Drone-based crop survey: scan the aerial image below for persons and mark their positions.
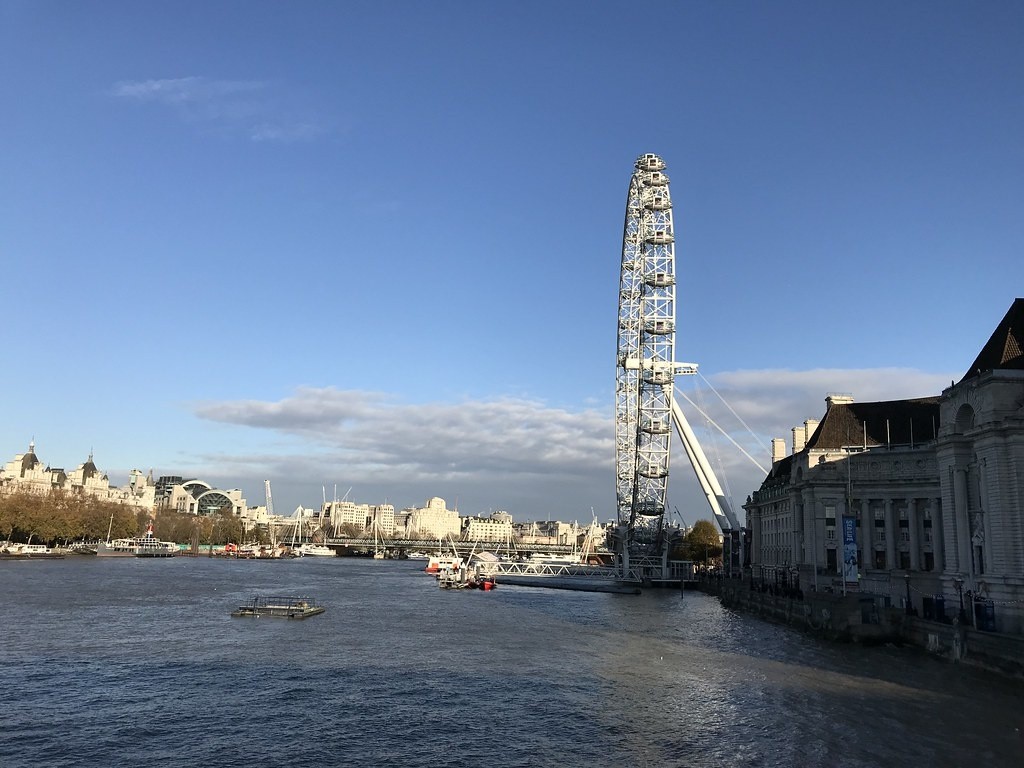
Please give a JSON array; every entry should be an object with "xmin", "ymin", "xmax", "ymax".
[{"xmin": 440, "ymin": 569, "xmax": 446, "ymax": 583}]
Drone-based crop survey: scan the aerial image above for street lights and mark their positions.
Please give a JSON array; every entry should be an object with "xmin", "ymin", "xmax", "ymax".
[
  {"xmin": 953, "ymin": 578, "xmax": 969, "ymax": 626},
  {"xmin": 748, "ymin": 564, "xmax": 794, "ymax": 599},
  {"xmin": 904, "ymin": 575, "xmax": 914, "ymax": 619}
]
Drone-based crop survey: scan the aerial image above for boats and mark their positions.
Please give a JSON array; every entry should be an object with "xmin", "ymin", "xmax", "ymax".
[
  {"xmin": 0, "ymin": 544, "xmax": 52, "ymax": 555},
  {"xmin": 425, "ymin": 554, "xmax": 464, "ymax": 575},
  {"xmin": 230, "ymin": 542, "xmax": 337, "ymax": 559},
  {"xmin": 97, "ymin": 537, "xmax": 181, "ymax": 557}
]
